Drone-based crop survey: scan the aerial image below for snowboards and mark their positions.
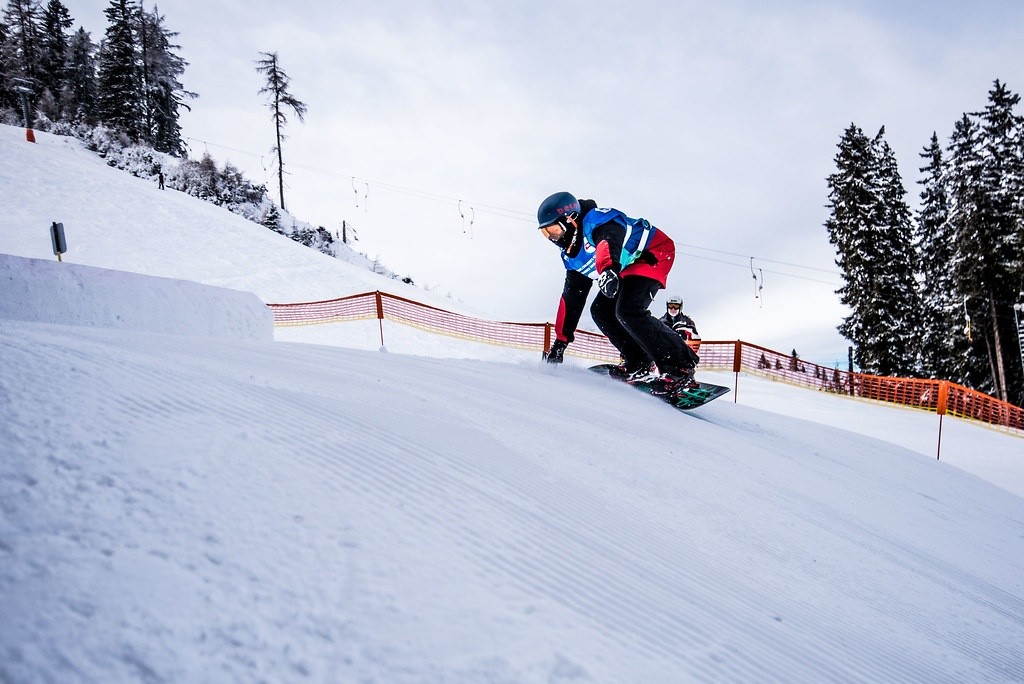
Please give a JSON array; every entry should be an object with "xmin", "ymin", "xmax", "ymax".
[{"xmin": 588, "ymin": 364, "xmax": 730, "ymax": 410}]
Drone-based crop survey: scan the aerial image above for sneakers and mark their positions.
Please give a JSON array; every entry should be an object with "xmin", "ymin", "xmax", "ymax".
[
  {"xmin": 610, "ymin": 362, "xmax": 655, "ymax": 384},
  {"xmin": 650, "ymin": 366, "xmax": 696, "ymax": 396}
]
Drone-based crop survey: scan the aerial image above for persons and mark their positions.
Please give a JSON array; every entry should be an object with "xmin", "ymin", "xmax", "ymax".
[
  {"xmin": 158, "ymin": 173, "xmax": 164, "ymax": 190},
  {"xmin": 536, "ymin": 191, "xmax": 700, "ymax": 398},
  {"xmin": 658, "ymin": 294, "xmax": 703, "ymax": 353}
]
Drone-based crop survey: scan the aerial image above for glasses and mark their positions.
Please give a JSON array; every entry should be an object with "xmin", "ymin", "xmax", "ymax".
[{"xmin": 668, "ymin": 304, "xmax": 680, "ymax": 309}]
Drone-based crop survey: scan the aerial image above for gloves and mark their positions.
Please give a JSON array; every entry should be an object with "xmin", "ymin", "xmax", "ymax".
[
  {"xmin": 597, "ymin": 269, "xmax": 619, "ymax": 298},
  {"xmin": 546, "ymin": 339, "xmax": 567, "ymax": 363}
]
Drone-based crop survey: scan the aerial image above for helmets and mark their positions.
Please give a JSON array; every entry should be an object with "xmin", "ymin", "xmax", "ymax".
[
  {"xmin": 665, "ymin": 296, "xmax": 683, "ymax": 315},
  {"xmin": 536, "ymin": 191, "xmax": 581, "ymax": 248}
]
